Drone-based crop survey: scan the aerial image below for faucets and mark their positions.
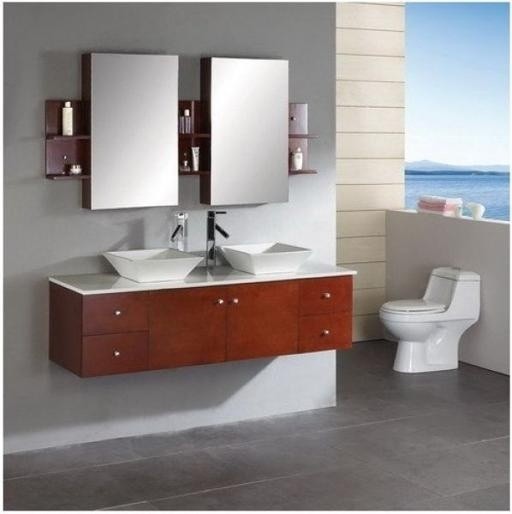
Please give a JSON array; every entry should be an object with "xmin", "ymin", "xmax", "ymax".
[
  {"xmin": 204, "ymin": 208, "xmax": 230, "ymax": 269},
  {"xmin": 171, "ymin": 210, "xmax": 190, "ymax": 251}
]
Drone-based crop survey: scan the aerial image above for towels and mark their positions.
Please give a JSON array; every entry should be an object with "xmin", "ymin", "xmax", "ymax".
[{"xmin": 415, "ymin": 194, "xmax": 463, "ymax": 219}]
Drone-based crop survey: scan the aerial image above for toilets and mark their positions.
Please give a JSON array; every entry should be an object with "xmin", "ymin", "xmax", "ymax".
[{"xmin": 377, "ymin": 268, "xmax": 481, "ymax": 374}]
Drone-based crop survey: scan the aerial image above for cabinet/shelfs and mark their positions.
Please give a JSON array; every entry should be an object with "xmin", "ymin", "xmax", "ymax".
[
  {"xmin": 47, "ymin": 276, "xmax": 354, "ymax": 378},
  {"xmin": 179, "ymin": 99, "xmax": 207, "ymax": 176},
  {"xmin": 289, "ymin": 102, "xmax": 318, "ymax": 175},
  {"xmin": 45, "ymin": 98, "xmax": 91, "ymax": 181}
]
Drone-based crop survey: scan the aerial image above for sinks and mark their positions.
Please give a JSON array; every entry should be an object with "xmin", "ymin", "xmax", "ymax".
[
  {"xmin": 102, "ymin": 246, "xmax": 204, "ymax": 285},
  {"xmin": 215, "ymin": 240, "xmax": 314, "ymax": 274}
]
